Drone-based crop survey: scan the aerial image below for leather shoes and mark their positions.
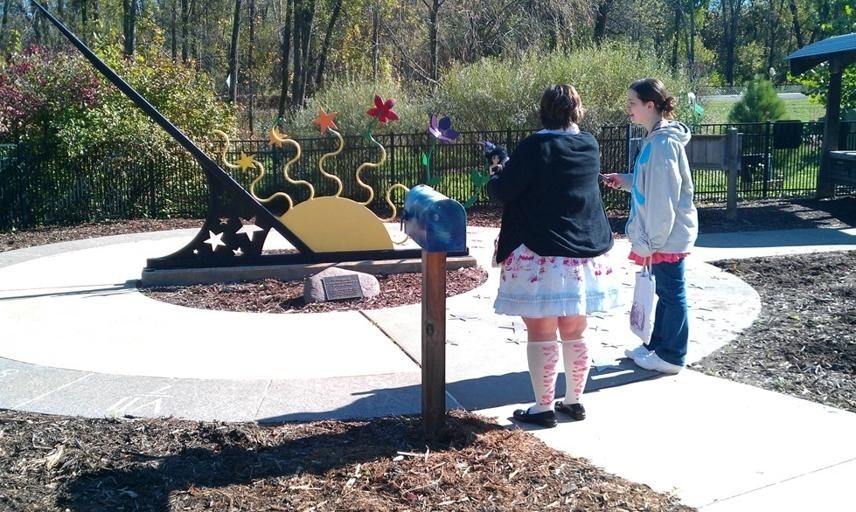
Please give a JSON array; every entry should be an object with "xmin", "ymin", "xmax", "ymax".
[
  {"xmin": 554, "ymin": 400, "xmax": 586, "ymax": 421},
  {"xmin": 513, "ymin": 408, "xmax": 558, "ymax": 428}
]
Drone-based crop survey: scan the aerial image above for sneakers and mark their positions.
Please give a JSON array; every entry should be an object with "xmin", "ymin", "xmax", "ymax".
[{"xmin": 624, "ymin": 345, "xmax": 684, "ymax": 375}]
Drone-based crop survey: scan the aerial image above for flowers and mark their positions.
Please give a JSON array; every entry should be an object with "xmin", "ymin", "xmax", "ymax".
[
  {"xmin": 418, "ymin": 112, "xmax": 462, "ymax": 188},
  {"xmin": 355, "ymin": 93, "xmax": 400, "ymax": 206}
]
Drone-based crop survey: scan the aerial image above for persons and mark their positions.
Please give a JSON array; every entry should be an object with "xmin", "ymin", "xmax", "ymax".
[
  {"xmin": 486, "ymin": 149, "xmax": 504, "ymax": 176},
  {"xmin": 485, "ymin": 82, "xmax": 617, "ymax": 427},
  {"xmin": 603, "ymin": 75, "xmax": 701, "ymax": 374}
]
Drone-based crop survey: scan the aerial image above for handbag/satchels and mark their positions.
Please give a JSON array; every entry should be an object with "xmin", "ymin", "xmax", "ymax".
[{"xmin": 629, "ymin": 255, "xmax": 657, "ymax": 346}]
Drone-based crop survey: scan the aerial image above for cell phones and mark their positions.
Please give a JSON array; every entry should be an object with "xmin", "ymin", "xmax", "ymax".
[{"xmin": 599, "ymin": 173, "xmax": 611, "ymax": 182}]
